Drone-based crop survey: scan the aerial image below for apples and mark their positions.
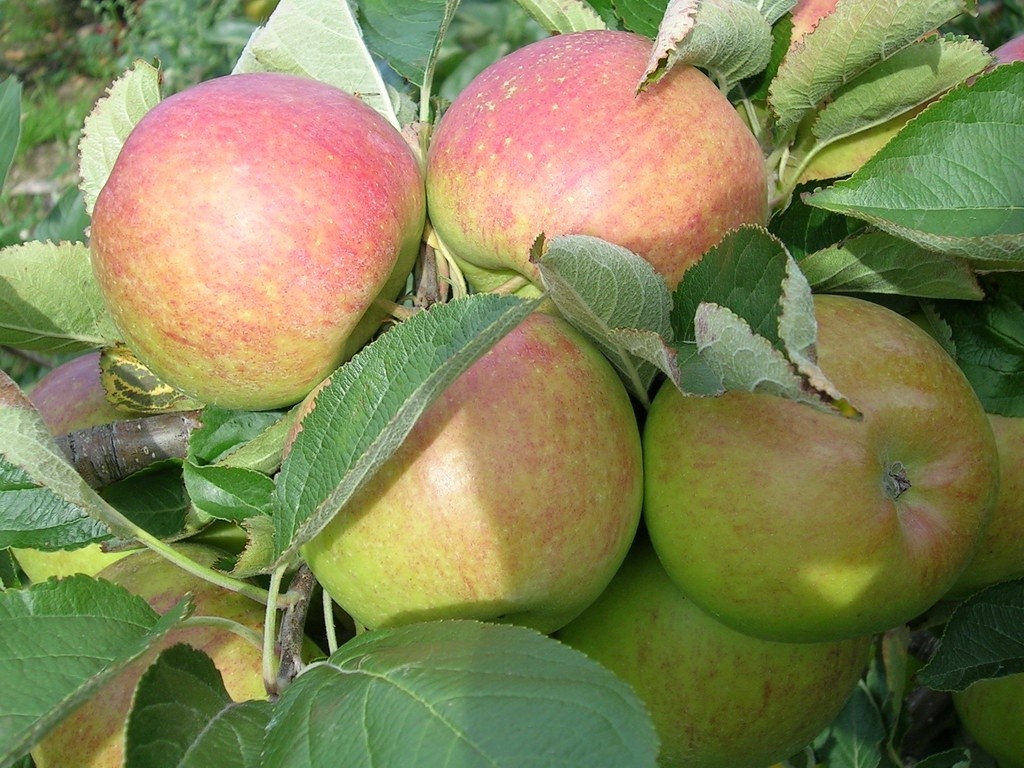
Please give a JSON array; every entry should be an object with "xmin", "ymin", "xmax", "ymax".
[{"xmin": 1, "ymin": 0, "xmax": 1024, "ymax": 768}]
